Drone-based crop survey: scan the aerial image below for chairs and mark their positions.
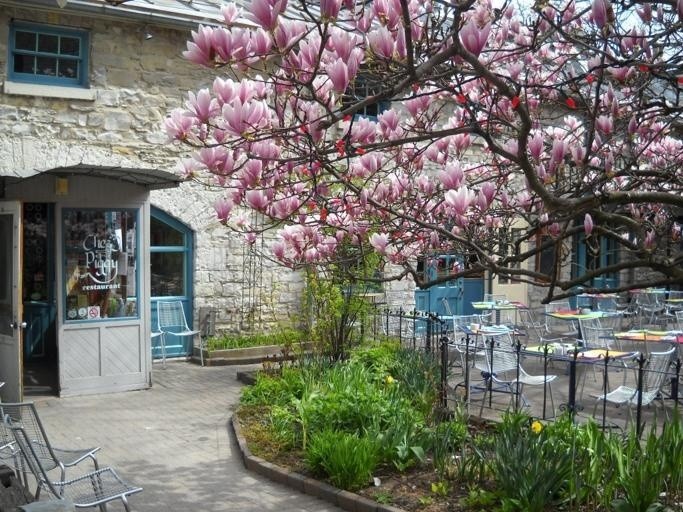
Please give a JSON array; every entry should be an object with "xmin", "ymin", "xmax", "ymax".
[
  {"xmin": 545, "ymin": 288, "xmax": 683, "ymax": 432},
  {"xmin": 439, "ymin": 294, "xmax": 582, "ymax": 418},
  {"xmin": 151, "ymin": 331, "xmax": 166, "ymax": 367},
  {"xmin": 157, "ymin": 299, "xmax": 204, "ymax": 368},
  {"xmin": 0, "ymin": 400, "xmax": 144, "ymax": 512}
]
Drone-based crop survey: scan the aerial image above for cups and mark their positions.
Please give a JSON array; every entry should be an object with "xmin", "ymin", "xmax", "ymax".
[{"xmin": 470, "ymin": 323, "xmax": 480, "ymax": 331}]
